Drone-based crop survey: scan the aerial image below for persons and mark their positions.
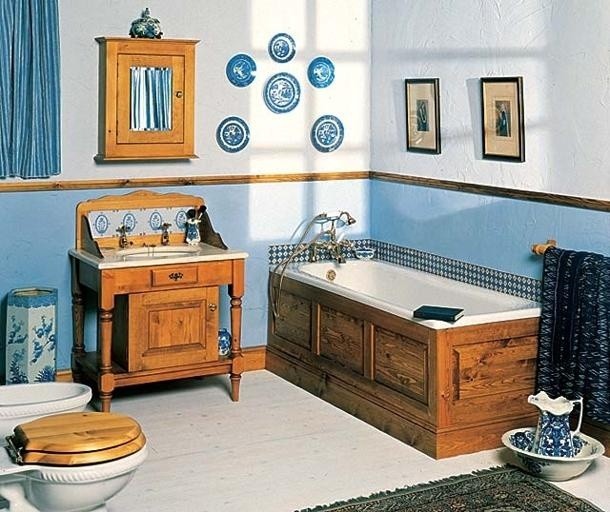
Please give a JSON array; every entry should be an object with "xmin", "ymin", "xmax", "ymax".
[
  {"xmin": 499, "ymin": 103, "xmax": 509, "ymax": 136},
  {"xmin": 419, "ymin": 102, "xmax": 427, "ymax": 131}
]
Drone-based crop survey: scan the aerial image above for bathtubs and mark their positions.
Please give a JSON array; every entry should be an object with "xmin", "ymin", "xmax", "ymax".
[{"xmin": 297, "ymin": 253, "xmax": 542, "ymax": 331}]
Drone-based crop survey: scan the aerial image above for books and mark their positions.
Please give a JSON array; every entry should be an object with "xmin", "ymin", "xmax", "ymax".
[{"xmin": 413, "ymin": 305, "xmax": 465, "ymax": 322}]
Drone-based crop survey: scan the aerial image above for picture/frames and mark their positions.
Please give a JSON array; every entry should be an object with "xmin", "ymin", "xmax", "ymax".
[
  {"xmin": 479, "ymin": 76, "xmax": 527, "ymax": 165},
  {"xmin": 403, "ymin": 77, "xmax": 442, "ymax": 155}
]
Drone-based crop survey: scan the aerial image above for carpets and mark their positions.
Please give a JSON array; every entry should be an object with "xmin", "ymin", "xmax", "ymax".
[{"xmin": 292, "ymin": 461, "xmax": 604, "ymax": 512}]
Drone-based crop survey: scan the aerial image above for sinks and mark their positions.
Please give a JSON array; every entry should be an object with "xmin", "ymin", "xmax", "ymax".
[
  {"xmin": 120, "ymin": 247, "xmax": 200, "ymax": 260},
  {"xmin": 0, "ymin": 381, "xmax": 93, "ymax": 442}
]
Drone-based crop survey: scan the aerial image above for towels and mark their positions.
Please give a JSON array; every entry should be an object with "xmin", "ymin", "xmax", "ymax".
[{"xmin": 534, "ymin": 245, "xmax": 610, "ymax": 434}]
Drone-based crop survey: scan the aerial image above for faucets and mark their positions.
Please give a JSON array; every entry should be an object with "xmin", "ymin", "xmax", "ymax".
[
  {"xmin": 116, "ymin": 225, "xmax": 127, "ymax": 248},
  {"xmin": 327, "ymin": 240, "xmax": 347, "ymax": 264},
  {"xmin": 158, "ymin": 222, "xmax": 172, "ymax": 244}
]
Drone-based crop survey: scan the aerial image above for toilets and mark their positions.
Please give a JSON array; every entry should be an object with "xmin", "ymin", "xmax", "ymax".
[{"xmin": 0, "ymin": 411, "xmax": 148, "ymax": 510}]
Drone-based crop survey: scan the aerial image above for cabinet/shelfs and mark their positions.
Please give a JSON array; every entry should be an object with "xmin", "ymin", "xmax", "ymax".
[
  {"xmin": 93, "ymin": 35, "xmax": 201, "ymax": 163},
  {"xmin": 70, "ymin": 257, "xmax": 246, "ymax": 413}
]
